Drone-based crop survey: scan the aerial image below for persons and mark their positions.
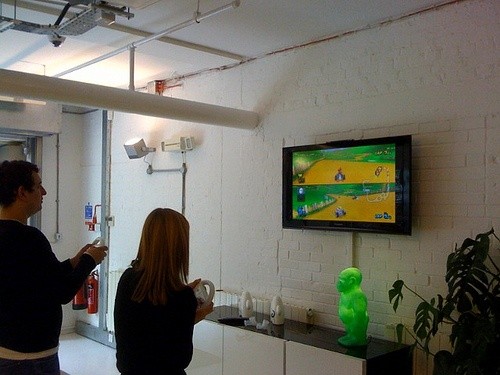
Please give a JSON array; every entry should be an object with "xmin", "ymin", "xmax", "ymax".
[
  {"xmin": 0, "ymin": 160, "xmax": 107, "ymax": 374},
  {"xmin": 335, "ymin": 267, "xmax": 370, "ymax": 346},
  {"xmin": 115, "ymin": 207, "xmax": 214, "ymax": 375}
]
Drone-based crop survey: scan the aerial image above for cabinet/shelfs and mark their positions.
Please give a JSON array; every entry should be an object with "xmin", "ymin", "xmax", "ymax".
[
  {"xmin": 223, "ymin": 323, "xmax": 287, "ymax": 375},
  {"xmin": 184, "ymin": 320, "xmax": 223, "ymax": 375},
  {"xmin": 285, "ymin": 340, "xmax": 364, "ymax": 375}
]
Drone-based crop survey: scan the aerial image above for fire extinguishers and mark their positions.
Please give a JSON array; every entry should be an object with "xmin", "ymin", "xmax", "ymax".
[{"xmin": 86, "ymin": 271, "xmax": 98, "ymax": 313}]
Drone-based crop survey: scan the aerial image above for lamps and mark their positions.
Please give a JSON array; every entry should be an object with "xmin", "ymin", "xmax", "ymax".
[{"xmin": 123, "ymin": 136, "xmax": 157, "ymax": 159}]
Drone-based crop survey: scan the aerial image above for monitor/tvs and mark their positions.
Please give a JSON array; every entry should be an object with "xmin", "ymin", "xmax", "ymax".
[{"xmin": 282, "ymin": 135, "xmax": 412, "ymax": 236}]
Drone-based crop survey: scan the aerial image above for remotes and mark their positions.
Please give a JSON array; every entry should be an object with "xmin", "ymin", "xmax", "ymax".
[{"xmin": 218, "ymin": 319, "xmax": 252, "ymax": 327}]
